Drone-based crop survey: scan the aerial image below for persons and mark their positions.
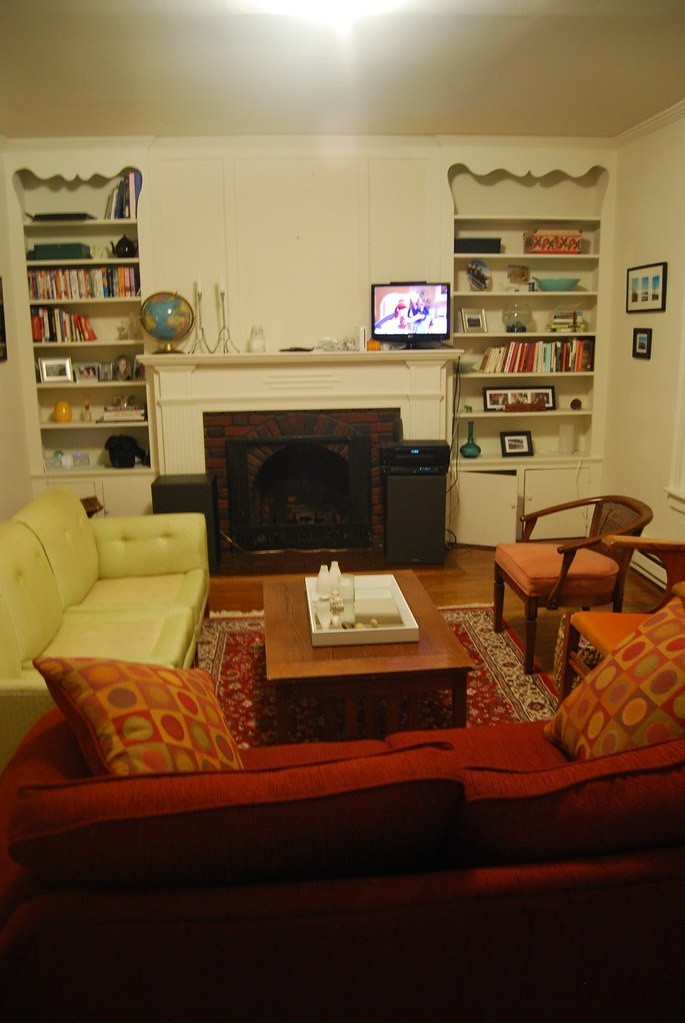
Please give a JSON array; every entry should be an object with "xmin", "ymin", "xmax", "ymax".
[{"xmin": 115, "ymin": 356, "xmax": 132, "ymax": 381}]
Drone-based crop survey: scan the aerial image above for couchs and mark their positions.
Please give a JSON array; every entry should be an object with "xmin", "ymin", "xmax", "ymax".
[
  {"xmin": 0, "ymin": 705, "xmax": 685, "ymax": 1023},
  {"xmin": 0, "ymin": 483, "xmax": 210, "ymax": 774}
]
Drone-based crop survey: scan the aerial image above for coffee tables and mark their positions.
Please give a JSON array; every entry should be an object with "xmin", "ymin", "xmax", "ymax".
[{"xmin": 261, "ymin": 569, "xmax": 473, "ymax": 744}]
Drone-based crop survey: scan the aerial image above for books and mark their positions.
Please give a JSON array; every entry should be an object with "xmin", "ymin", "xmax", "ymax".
[
  {"xmin": 485, "ymin": 341, "xmax": 583, "ymax": 373},
  {"xmin": 27, "ymin": 266, "xmax": 135, "ymax": 299},
  {"xmin": 31, "ymin": 306, "xmax": 97, "ymax": 343},
  {"xmin": 104, "ymin": 174, "xmax": 129, "ymax": 220},
  {"xmin": 545, "ymin": 311, "xmax": 585, "ymax": 332}
]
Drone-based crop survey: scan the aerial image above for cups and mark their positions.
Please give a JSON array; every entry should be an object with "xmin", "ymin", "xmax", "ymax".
[{"xmin": 338, "ymin": 574, "xmax": 355, "ymax": 601}]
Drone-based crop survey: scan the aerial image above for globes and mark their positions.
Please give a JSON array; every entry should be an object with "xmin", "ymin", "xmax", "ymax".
[{"xmin": 136, "ymin": 290, "xmax": 194, "ymax": 354}]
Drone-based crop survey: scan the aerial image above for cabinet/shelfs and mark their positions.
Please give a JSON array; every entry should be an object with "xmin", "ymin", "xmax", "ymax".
[
  {"xmin": 452, "ymin": 212, "xmax": 602, "ymax": 467},
  {"xmin": 23, "ymin": 218, "xmax": 158, "ymax": 477},
  {"xmin": 32, "ymin": 475, "xmax": 155, "ymax": 518},
  {"xmin": 450, "ymin": 466, "xmax": 592, "ymax": 547}
]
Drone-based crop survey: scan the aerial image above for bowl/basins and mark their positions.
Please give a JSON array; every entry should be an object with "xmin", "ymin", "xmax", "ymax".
[{"xmin": 536, "ymin": 278, "xmax": 581, "ymax": 291}]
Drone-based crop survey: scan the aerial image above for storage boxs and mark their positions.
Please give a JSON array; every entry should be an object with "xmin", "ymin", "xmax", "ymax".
[
  {"xmin": 522, "ymin": 228, "xmax": 584, "ymax": 254},
  {"xmin": 34, "ymin": 243, "xmax": 91, "ymax": 260}
]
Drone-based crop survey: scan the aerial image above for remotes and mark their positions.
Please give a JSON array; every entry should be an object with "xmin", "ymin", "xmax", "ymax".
[{"xmin": 280, "ymin": 346, "xmax": 314, "ymax": 352}]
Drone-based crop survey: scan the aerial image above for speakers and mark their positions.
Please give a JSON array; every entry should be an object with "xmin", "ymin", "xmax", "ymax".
[
  {"xmin": 387, "ymin": 476, "xmax": 447, "ymax": 566},
  {"xmin": 151, "ymin": 474, "xmax": 225, "ymax": 575}
]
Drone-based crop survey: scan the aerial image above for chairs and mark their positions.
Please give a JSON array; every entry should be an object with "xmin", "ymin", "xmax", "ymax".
[
  {"xmin": 556, "ymin": 534, "xmax": 685, "ymax": 708},
  {"xmin": 492, "ymin": 493, "xmax": 653, "ymax": 675}
]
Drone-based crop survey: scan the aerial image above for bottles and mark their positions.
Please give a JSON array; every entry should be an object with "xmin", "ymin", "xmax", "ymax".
[
  {"xmin": 330, "ymin": 561, "xmax": 342, "ymax": 595},
  {"xmin": 250, "ymin": 326, "xmax": 265, "ymax": 352},
  {"xmin": 502, "ymin": 302, "xmax": 533, "ymax": 332},
  {"xmin": 460, "ymin": 421, "xmax": 481, "ymax": 458},
  {"xmin": 316, "ymin": 565, "xmax": 331, "ymax": 601},
  {"xmin": 120, "ymin": 312, "xmax": 137, "ymax": 340},
  {"xmin": 330, "ymin": 590, "xmax": 343, "ymax": 609},
  {"xmin": 53, "ymin": 450, "xmax": 81, "ymax": 471},
  {"xmin": 54, "ymin": 401, "xmax": 72, "ymax": 423}
]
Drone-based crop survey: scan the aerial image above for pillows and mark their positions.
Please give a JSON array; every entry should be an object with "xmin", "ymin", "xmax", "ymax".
[
  {"xmin": 540, "ymin": 595, "xmax": 685, "ymax": 763},
  {"xmin": 32, "ymin": 655, "xmax": 243, "ymax": 776}
]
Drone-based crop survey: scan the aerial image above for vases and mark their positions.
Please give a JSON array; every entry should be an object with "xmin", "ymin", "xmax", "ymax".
[{"xmin": 460, "ymin": 421, "xmax": 481, "ymax": 458}]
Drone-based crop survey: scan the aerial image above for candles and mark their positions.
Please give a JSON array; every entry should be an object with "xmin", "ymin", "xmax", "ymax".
[
  {"xmin": 218, "ymin": 278, "xmax": 226, "ymax": 294},
  {"xmin": 195, "ymin": 279, "xmax": 202, "ymax": 294}
]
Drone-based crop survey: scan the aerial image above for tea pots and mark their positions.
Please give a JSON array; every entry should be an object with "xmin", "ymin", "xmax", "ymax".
[{"xmin": 110, "ymin": 234, "xmax": 139, "ymax": 258}]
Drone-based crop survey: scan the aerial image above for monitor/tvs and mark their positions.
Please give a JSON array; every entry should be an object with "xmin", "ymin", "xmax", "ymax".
[{"xmin": 372, "ymin": 282, "xmax": 451, "ymax": 350}]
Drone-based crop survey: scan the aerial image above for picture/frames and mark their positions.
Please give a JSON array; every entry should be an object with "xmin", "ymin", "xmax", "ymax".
[
  {"xmin": 499, "ymin": 429, "xmax": 535, "ymax": 458},
  {"xmin": 632, "ymin": 327, "xmax": 652, "ymax": 359},
  {"xmin": 482, "ymin": 386, "xmax": 558, "ymax": 412},
  {"xmin": 626, "ymin": 261, "xmax": 668, "ymax": 314},
  {"xmin": 460, "ymin": 307, "xmax": 487, "ymax": 333},
  {"xmin": 99, "ymin": 361, "xmax": 113, "ymax": 381},
  {"xmin": 73, "ymin": 361, "xmax": 99, "ymax": 383},
  {"xmin": 37, "ymin": 355, "xmax": 75, "ymax": 384}
]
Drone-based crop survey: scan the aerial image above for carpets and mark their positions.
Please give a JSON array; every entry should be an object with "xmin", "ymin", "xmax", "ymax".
[{"xmin": 194, "ymin": 601, "xmax": 563, "ymax": 749}]
